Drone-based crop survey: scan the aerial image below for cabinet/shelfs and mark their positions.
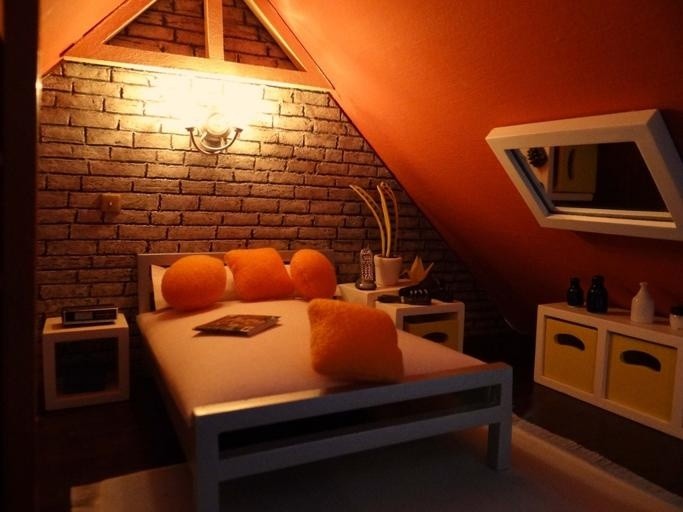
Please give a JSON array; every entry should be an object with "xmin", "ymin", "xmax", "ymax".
[
  {"xmin": 512, "ymin": 146, "xmax": 600, "ymax": 203},
  {"xmin": 533, "ymin": 302, "xmax": 683, "ymax": 438}
]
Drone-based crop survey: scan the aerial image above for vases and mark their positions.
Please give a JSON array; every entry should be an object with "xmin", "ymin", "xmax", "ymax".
[
  {"xmin": 567, "ymin": 277, "xmax": 584, "ymax": 306},
  {"xmin": 586, "ymin": 275, "xmax": 608, "ymax": 314}
]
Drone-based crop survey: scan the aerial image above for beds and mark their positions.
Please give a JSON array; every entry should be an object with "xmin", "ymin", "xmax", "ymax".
[{"xmin": 136, "ymin": 251, "xmax": 513, "ymax": 512}]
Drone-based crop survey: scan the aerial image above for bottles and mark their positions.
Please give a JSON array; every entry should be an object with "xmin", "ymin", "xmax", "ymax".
[{"xmin": 566, "ymin": 274, "xmax": 609, "ymax": 315}]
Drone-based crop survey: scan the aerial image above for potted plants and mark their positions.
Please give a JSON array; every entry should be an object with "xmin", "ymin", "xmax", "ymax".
[{"xmin": 348, "ymin": 182, "xmax": 403, "ymax": 286}]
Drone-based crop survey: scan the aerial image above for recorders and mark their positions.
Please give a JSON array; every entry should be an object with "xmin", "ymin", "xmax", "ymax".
[{"xmin": 61, "ymin": 302, "xmax": 118, "ymax": 326}]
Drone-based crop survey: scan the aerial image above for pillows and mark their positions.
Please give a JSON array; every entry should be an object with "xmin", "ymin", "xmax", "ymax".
[
  {"xmin": 308, "ymin": 297, "xmax": 404, "ymax": 380},
  {"xmin": 225, "ymin": 246, "xmax": 294, "ymax": 303},
  {"xmin": 150, "ymin": 264, "xmax": 343, "ymax": 310},
  {"xmin": 162, "ymin": 256, "xmax": 226, "ymax": 311},
  {"xmin": 290, "ymin": 248, "xmax": 337, "ymax": 301}
]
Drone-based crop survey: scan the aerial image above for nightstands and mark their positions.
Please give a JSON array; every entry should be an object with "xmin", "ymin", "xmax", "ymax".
[
  {"xmin": 338, "ymin": 279, "xmax": 465, "ymax": 354},
  {"xmin": 41, "ymin": 313, "xmax": 129, "ymax": 411}
]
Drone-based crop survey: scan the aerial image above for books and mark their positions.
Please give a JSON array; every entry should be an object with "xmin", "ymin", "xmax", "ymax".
[{"xmin": 191, "ymin": 314, "xmax": 281, "ymax": 338}]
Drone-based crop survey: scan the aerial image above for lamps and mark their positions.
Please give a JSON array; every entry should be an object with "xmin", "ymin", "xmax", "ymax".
[{"xmin": 184, "ymin": 111, "xmax": 244, "ymax": 154}]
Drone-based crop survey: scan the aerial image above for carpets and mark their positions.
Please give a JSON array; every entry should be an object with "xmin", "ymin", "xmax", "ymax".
[{"xmin": 71, "ymin": 407, "xmax": 683, "ymax": 512}]
